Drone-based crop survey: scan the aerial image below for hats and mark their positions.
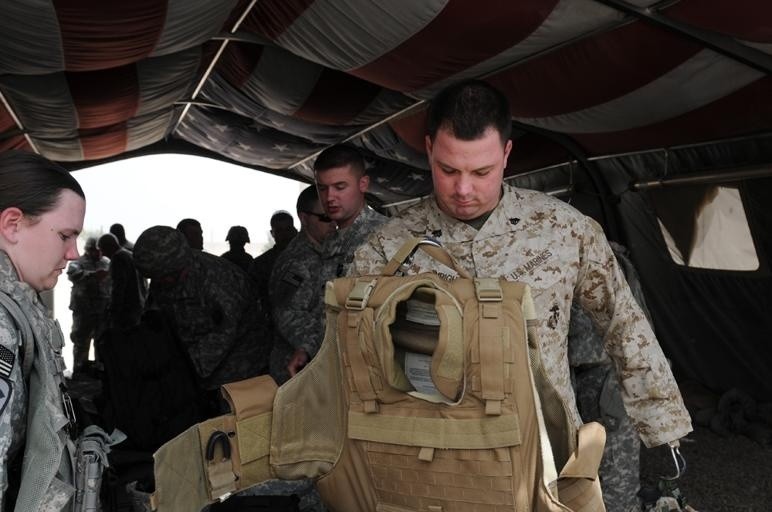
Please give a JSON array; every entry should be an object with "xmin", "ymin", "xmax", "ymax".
[
  {"xmin": 85, "ymin": 238, "xmax": 99, "ymax": 250},
  {"xmin": 132, "ymin": 226, "xmax": 194, "ymax": 276},
  {"xmin": 226, "ymin": 228, "xmax": 250, "ymax": 244}
]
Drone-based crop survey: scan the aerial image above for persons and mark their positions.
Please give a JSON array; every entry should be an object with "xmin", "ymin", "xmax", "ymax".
[
  {"xmin": 343, "ymin": 87, "xmax": 693, "ymax": 512},
  {"xmin": 67, "ymin": 236, "xmax": 112, "ymax": 383},
  {"xmin": 248, "ymin": 210, "xmax": 297, "ymax": 282},
  {"xmin": 218, "ymin": 225, "xmax": 253, "ymax": 270},
  {"xmin": 110, "ymin": 224, "xmax": 134, "ymax": 252},
  {"xmin": 96, "ymin": 234, "xmax": 148, "ymax": 356},
  {"xmin": 0, "ymin": 147, "xmax": 86, "ymax": 512},
  {"xmin": 132, "ymin": 225, "xmax": 264, "ymax": 391},
  {"xmin": 567, "ymin": 237, "xmax": 652, "ymax": 512},
  {"xmin": 278, "ymin": 145, "xmax": 391, "ymax": 377},
  {"xmin": 177, "ymin": 218, "xmax": 203, "ymax": 251},
  {"xmin": 267, "ymin": 184, "xmax": 337, "ymax": 381}
]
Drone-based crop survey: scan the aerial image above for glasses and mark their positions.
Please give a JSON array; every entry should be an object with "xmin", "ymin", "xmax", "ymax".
[{"xmin": 302, "ymin": 210, "xmax": 334, "ymax": 226}]
